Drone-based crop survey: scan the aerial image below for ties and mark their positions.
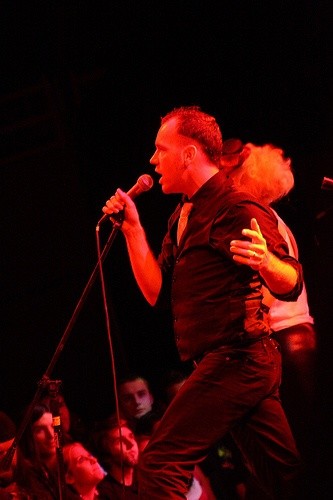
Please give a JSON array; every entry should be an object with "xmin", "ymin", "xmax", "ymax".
[{"xmin": 176, "ymin": 202, "xmax": 192, "ymax": 247}]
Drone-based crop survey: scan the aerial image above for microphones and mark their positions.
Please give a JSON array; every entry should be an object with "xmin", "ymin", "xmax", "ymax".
[{"xmin": 98, "ymin": 174, "xmax": 153, "ymax": 225}]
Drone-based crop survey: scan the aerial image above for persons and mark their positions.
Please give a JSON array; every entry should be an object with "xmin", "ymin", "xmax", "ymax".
[
  {"xmin": 0, "ymin": 373, "xmax": 248, "ymax": 500},
  {"xmin": 101, "ymin": 106, "xmax": 304, "ymax": 500},
  {"xmin": 221, "ymin": 142, "xmax": 320, "ymax": 439}
]
